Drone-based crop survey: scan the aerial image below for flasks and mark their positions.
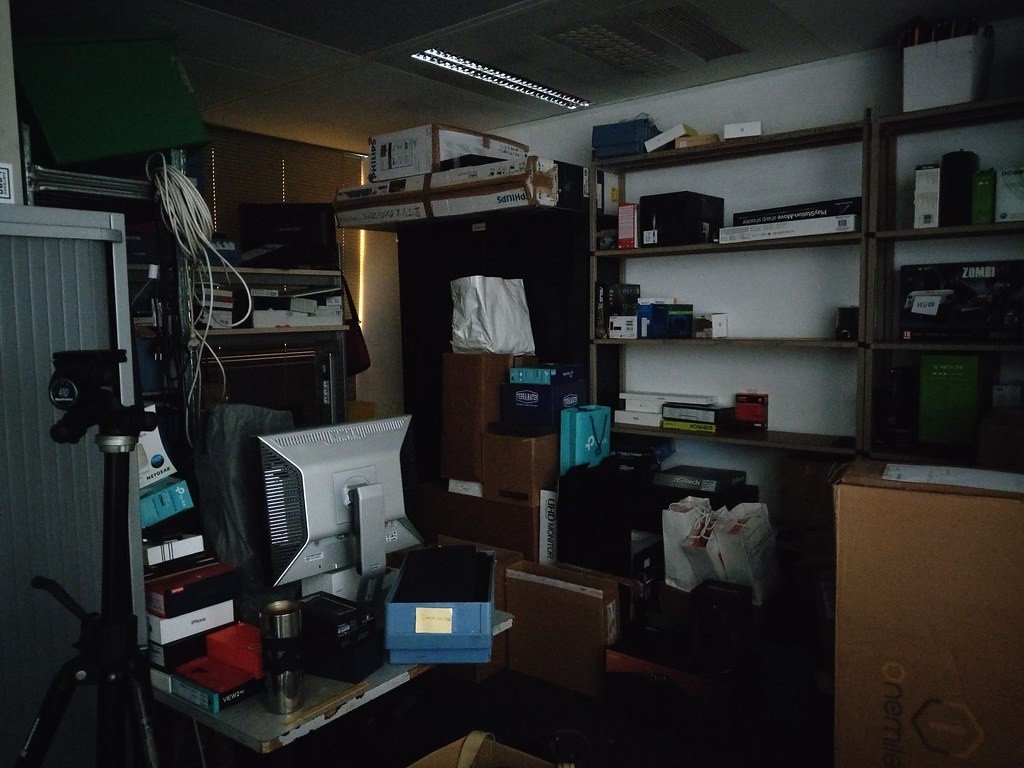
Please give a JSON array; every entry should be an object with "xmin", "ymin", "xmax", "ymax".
[{"xmin": 261, "ymin": 599, "xmax": 303, "ymax": 715}]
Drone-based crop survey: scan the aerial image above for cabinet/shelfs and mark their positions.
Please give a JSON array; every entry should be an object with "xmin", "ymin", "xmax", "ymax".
[
  {"xmin": 586, "ymin": 108, "xmax": 871, "ymax": 457},
  {"xmin": 868, "ymin": 92, "xmax": 1024, "ymax": 467}
]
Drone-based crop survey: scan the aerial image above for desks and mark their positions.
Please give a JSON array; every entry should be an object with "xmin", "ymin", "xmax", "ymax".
[{"xmin": 148, "ymin": 607, "xmax": 517, "ymax": 755}]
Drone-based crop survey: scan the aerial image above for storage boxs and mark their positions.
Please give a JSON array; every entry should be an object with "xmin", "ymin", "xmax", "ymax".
[
  {"xmin": 652, "ymin": 466, "xmax": 747, "ymax": 495},
  {"xmin": 423, "ymin": 488, "xmax": 540, "ymax": 563},
  {"xmin": 384, "ymin": 547, "xmax": 497, "ymax": 665},
  {"xmin": 436, "ymin": 533, "xmax": 523, "ymax": 674},
  {"xmin": 486, "ymin": 360, "xmax": 589, "ymax": 439},
  {"xmin": 971, "ymin": 165, "xmax": 996, "ymax": 226},
  {"xmin": 482, "ymin": 431, "xmax": 560, "ymax": 510},
  {"xmin": 606, "ymin": 623, "xmax": 762, "ymax": 755},
  {"xmin": 536, "ymin": 486, "xmax": 559, "ymax": 564},
  {"xmin": 334, "ymin": 156, "xmax": 619, "ymax": 230},
  {"xmin": 592, "ymin": 111, "xmax": 862, "ymax": 435},
  {"xmin": 830, "ymin": 458, "xmax": 1024, "ymax": 768},
  {"xmin": 684, "ymin": 579, "xmax": 754, "ymax": 652},
  {"xmin": 406, "ymin": 731, "xmax": 555, "ymax": 768},
  {"xmin": 995, "ymin": 166, "xmax": 1024, "ymax": 222},
  {"xmin": 438, "ymin": 352, "xmax": 540, "ymax": 483},
  {"xmin": 136, "ymin": 396, "xmax": 388, "ymax": 713},
  {"xmin": 503, "ymin": 558, "xmax": 621, "ymax": 700},
  {"xmin": 913, "ymin": 163, "xmax": 941, "ymax": 227},
  {"xmin": 899, "ymin": 260, "xmax": 1024, "ymax": 344},
  {"xmin": 367, "ymin": 122, "xmax": 530, "ymax": 184}
]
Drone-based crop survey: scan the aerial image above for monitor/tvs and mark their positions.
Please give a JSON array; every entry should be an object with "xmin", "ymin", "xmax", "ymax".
[{"xmin": 248, "ymin": 407, "xmax": 443, "ymax": 628}]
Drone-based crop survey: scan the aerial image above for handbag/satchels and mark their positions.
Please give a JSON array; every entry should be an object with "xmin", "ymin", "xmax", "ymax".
[
  {"xmin": 662, "ymin": 495, "xmax": 777, "ymax": 605},
  {"xmin": 560, "ymin": 404, "xmax": 611, "ymax": 477},
  {"xmin": 500, "ymin": 383, "xmax": 560, "ymax": 438},
  {"xmin": 450, "ymin": 275, "xmax": 535, "ymax": 357}
]
